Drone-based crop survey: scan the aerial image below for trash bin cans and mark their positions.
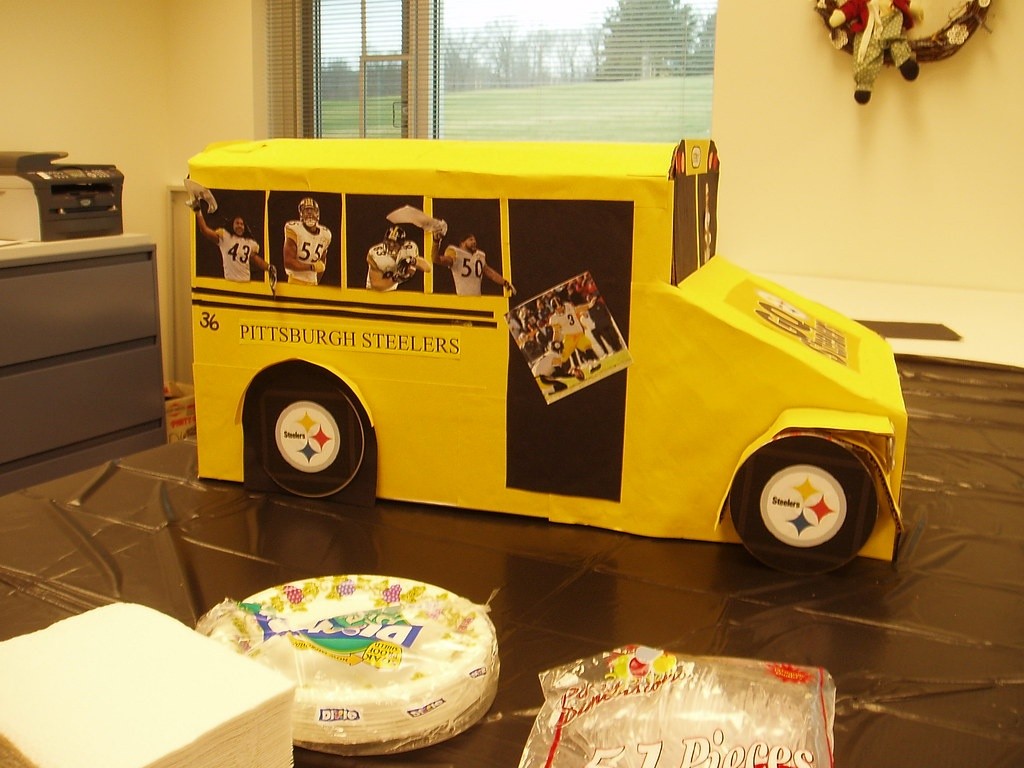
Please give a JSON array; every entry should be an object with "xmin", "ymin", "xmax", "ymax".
[
  {"xmin": 163, "ymin": 378, "xmax": 195, "ymax": 444},
  {"xmin": 641, "ymin": 55, "xmax": 652, "ymax": 78}
]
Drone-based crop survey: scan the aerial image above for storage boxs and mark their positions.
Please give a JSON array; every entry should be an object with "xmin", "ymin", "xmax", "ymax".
[{"xmin": 164, "ymin": 381, "xmax": 197, "ymax": 443}]
